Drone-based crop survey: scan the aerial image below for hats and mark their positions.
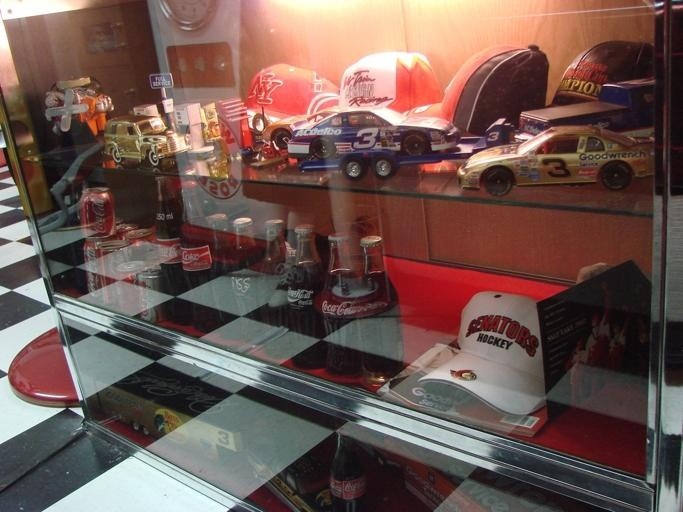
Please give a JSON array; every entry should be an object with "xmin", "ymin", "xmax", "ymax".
[
  {"xmin": 401, "ymin": 41, "xmax": 551, "ymax": 140},
  {"xmin": 418, "ymin": 289, "xmax": 546, "ymax": 418},
  {"xmin": 304, "ymin": 48, "xmax": 446, "ymax": 126},
  {"xmin": 545, "ymin": 38, "xmax": 655, "ymax": 110},
  {"xmin": 243, "ymin": 39, "xmax": 341, "ymax": 136}
]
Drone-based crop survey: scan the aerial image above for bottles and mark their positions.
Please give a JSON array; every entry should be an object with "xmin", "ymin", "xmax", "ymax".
[
  {"xmin": 317, "ymin": 231, "xmax": 362, "ymax": 374},
  {"xmin": 257, "ymin": 219, "xmax": 298, "ymax": 360},
  {"xmin": 230, "ymin": 215, "xmax": 266, "ymax": 346},
  {"xmin": 206, "ymin": 213, "xmax": 242, "ymax": 338},
  {"xmin": 178, "ymin": 179, "xmax": 222, "ymax": 336},
  {"xmin": 351, "ymin": 235, "xmax": 403, "ymax": 392},
  {"xmin": 286, "ymin": 224, "xmax": 326, "ymax": 369},
  {"xmin": 156, "ymin": 175, "xmax": 190, "ymax": 326},
  {"xmin": 329, "ymin": 430, "xmax": 372, "ymax": 511}
]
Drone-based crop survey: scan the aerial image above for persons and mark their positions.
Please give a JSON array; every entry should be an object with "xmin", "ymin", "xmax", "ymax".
[{"xmin": 567, "ymin": 281, "xmax": 631, "ymax": 402}]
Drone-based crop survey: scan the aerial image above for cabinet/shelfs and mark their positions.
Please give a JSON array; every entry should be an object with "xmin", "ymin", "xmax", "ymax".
[{"xmin": 0, "ymin": 1, "xmax": 683, "ymax": 511}]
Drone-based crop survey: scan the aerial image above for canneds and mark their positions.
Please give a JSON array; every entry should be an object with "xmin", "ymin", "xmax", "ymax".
[{"xmin": 80, "ymin": 186, "xmax": 162, "ymax": 323}]
